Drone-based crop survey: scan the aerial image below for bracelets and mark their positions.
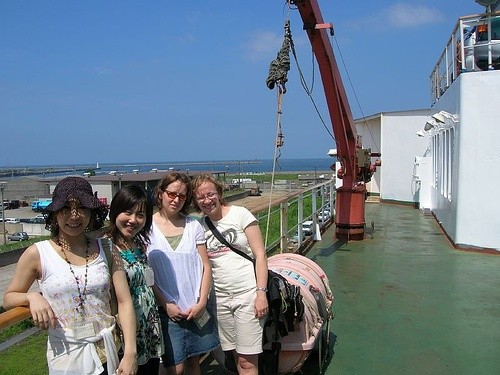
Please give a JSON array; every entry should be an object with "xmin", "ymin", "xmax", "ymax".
[{"xmin": 256, "ymin": 288, "xmax": 268, "ymax": 292}]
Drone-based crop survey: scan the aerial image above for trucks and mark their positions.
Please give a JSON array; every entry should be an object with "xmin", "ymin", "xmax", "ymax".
[{"xmin": 31, "ymin": 199, "xmax": 53, "ymax": 212}]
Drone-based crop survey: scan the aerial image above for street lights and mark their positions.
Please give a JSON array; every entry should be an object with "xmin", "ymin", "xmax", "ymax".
[{"xmin": 0, "ymin": 182, "xmax": 8, "ymax": 245}]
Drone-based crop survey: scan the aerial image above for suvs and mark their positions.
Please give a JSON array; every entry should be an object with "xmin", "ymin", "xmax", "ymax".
[
  {"xmin": 30, "ymin": 215, "xmax": 48, "ymax": 222},
  {"xmin": 5, "ymin": 218, "xmax": 20, "ymax": 224},
  {"xmin": 7, "ymin": 231, "xmax": 29, "ymax": 242}
]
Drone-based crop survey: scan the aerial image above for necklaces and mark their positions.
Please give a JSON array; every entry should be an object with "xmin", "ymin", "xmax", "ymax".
[
  {"xmin": 58, "ymin": 235, "xmax": 90, "ymax": 311},
  {"xmin": 121, "ymin": 237, "xmax": 135, "ymax": 253}
]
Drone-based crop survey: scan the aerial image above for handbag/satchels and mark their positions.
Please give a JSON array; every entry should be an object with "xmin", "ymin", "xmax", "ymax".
[{"xmin": 252, "ymin": 258, "xmax": 304, "ymax": 337}]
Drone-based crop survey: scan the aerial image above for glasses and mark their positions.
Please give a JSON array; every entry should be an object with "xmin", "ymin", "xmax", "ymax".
[
  {"xmin": 196, "ymin": 192, "xmax": 218, "ymax": 202},
  {"xmin": 61, "ymin": 206, "xmax": 87, "ymax": 215}
]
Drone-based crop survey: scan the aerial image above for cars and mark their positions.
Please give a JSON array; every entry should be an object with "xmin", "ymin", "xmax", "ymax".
[{"xmin": 0, "ymin": 199, "xmax": 28, "ymax": 210}]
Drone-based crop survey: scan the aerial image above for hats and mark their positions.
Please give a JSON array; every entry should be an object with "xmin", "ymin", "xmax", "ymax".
[
  {"xmin": 162, "ymin": 189, "xmax": 187, "ymax": 201},
  {"xmin": 41, "ymin": 177, "xmax": 109, "ymax": 232}
]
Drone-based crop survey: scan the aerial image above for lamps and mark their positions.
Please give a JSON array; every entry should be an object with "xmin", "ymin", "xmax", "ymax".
[
  {"xmin": 416, "ymin": 130, "xmax": 432, "ymax": 137},
  {"xmin": 424, "ymin": 120, "xmax": 445, "ymax": 132},
  {"xmin": 432, "ymin": 110, "xmax": 459, "ymax": 125}
]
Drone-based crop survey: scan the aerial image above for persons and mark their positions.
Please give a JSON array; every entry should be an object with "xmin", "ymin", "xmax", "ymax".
[
  {"xmin": 190, "ymin": 173, "xmax": 269, "ymax": 375},
  {"xmin": 99, "ymin": 184, "xmax": 165, "ymax": 375},
  {"xmin": 140, "ymin": 170, "xmax": 220, "ymax": 375},
  {"xmin": 3, "ymin": 177, "xmax": 138, "ymax": 375}
]
